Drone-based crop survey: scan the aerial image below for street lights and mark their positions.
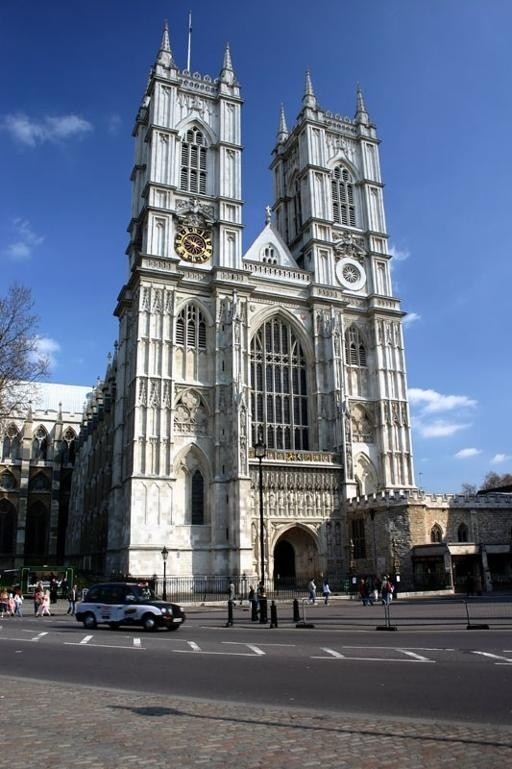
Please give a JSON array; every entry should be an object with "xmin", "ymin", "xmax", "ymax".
[
  {"xmin": 160, "ymin": 545, "xmax": 169, "ymax": 602},
  {"xmin": 252, "ymin": 434, "xmax": 271, "ymax": 592},
  {"xmin": 347, "ymin": 538, "xmax": 357, "ymax": 597}
]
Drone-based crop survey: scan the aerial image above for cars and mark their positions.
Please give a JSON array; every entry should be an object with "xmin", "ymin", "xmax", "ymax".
[{"xmin": 72, "ymin": 581, "xmax": 187, "ymax": 634}]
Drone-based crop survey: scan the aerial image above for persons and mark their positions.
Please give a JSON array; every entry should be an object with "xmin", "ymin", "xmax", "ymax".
[
  {"xmin": 358, "ymin": 574, "xmax": 395, "ymax": 608},
  {"xmin": 142, "ymin": 581, "xmax": 151, "ymax": 599},
  {"xmin": 0, "ymin": 576, "xmax": 78, "ymax": 618},
  {"xmin": 257, "ymin": 581, "xmax": 266, "ymax": 613},
  {"xmin": 248, "ymin": 585, "xmax": 255, "ymax": 599},
  {"xmin": 227, "ymin": 579, "xmax": 237, "ymax": 608},
  {"xmin": 322, "ymin": 579, "xmax": 332, "ymax": 605},
  {"xmin": 307, "ymin": 577, "xmax": 318, "ymax": 605}
]
